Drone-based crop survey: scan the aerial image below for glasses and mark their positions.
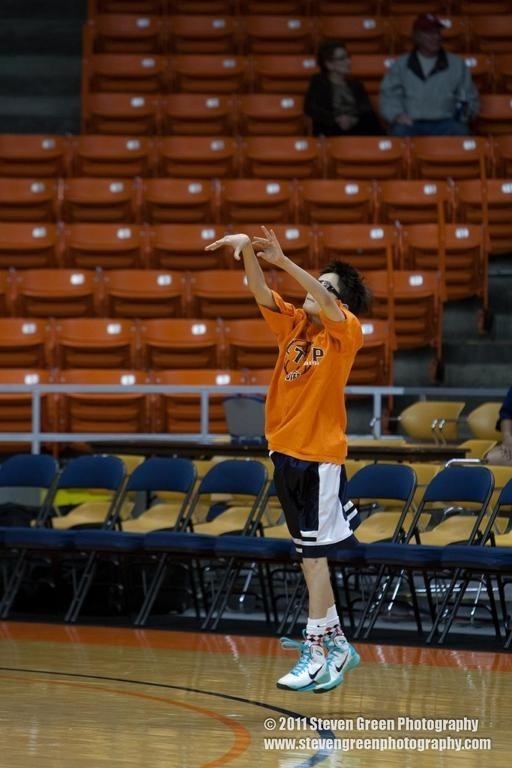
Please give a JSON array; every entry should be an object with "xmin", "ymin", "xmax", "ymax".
[
  {"xmin": 331, "ymin": 52, "xmax": 352, "ymax": 62},
  {"xmin": 318, "ymin": 279, "xmax": 343, "ymax": 300}
]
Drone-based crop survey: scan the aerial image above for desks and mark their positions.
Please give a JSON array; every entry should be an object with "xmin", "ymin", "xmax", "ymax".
[{"xmin": 84, "ymin": 440, "xmax": 472, "ymax": 464}]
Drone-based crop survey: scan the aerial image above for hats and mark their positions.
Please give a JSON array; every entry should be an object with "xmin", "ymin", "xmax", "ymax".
[{"xmin": 413, "ymin": 11, "xmax": 446, "ymax": 31}]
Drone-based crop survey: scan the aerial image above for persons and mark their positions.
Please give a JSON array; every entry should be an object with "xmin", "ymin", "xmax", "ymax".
[
  {"xmin": 378, "ymin": 13, "xmax": 482, "ymax": 136},
  {"xmin": 205, "ymin": 225, "xmax": 364, "ymax": 694},
  {"xmin": 485, "ymin": 387, "xmax": 512, "ymax": 467},
  {"xmin": 302, "ymin": 39, "xmax": 374, "ymax": 136}
]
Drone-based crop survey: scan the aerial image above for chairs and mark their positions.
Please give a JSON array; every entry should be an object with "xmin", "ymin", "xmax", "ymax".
[
  {"xmin": 139, "ymin": 180, "xmax": 219, "ymax": 224},
  {"xmin": 408, "ymin": 465, "xmax": 511, "ymax": 545},
  {"xmin": 156, "ymin": 136, "xmax": 242, "ymax": 178},
  {"xmin": 62, "ymin": 179, "xmax": 139, "ymax": 222},
  {"xmin": 72, "ymin": 457, "xmax": 203, "ymax": 626},
  {"xmin": 55, "ymin": 370, "xmax": 153, "ymax": 456},
  {"xmin": 137, "ymin": 319, "xmax": 224, "ymax": 371},
  {"xmin": 354, "ymin": 462, "xmax": 442, "ymax": 544},
  {"xmin": 188, "ymin": 271, "xmax": 276, "ymax": 319},
  {"xmin": 489, "ymin": 136, "xmax": 511, "ymax": 179},
  {"xmin": 88, "ymin": 15, "xmax": 163, "ymax": 54},
  {"xmin": 275, "ymin": 272, "xmax": 323, "ymax": 319},
  {"xmin": 0, "ymin": 135, "xmax": 69, "ymax": 177},
  {"xmin": 0, "ymin": 453, "xmax": 58, "ymax": 618},
  {"xmin": 238, "ymin": 15, "xmax": 317, "ymax": 55},
  {"xmin": 346, "ymin": 54, "xmax": 399, "ymax": 92},
  {"xmin": 161, "ymin": 94, "xmax": 241, "ymax": 136},
  {"xmin": 211, "ymin": 478, "xmax": 345, "ymax": 636},
  {"xmin": 82, "ymin": 95, "xmax": 162, "ymax": 136},
  {"xmin": 115, "ymin": 460, "xmax": 216, "ymax": 535},
  {"xmin": 232, "ymin": 224, "xmax": 316, "ymax": 267},
  {"xmin": 140, "ymin": 459, "xmax": 272, "ymax": 633},
  {"xmin": 407, "ymin": 136, "xmax": 495, "ymax": 180},
  {"xmin": 322, "ymin": 136, "xmax": 410, "ymax": 179},
  {"xmin": 219, "ymin": 180, "xmax": 297, "ymax": 223},
  {"xmin": 68, "ymin": 134, "xmax": 157, "ymax": 178},
  {"xmin": 13, "ymin": 269, "xmax": 101, "ymax": 318},
  {"xmin": 184, "ymin": 455, "xmax": 275, "ymax": 538},
  {"xmin": 0, "ymin": 178, "xmax": 62, "ymax": 222},
  {"xmin": 81, "ymin": 53, "xmax": 169, "ymax": 94},
  {"xmin": 285, "ymin": 464, "xmax": 424, "ymax": 639},
  {"xmin": 359, "ymin": 270, "xmax": 441, "ymax": 384},
  {"xmin": 438, "ymin": 477, "xmax": 512, "ymax": 650},
  {"xmin": 239, "ymin": 92, "xmax": 307, "ymax": 135},
  {"xmin": 220, "ymin": 319, "xmax": 279, "ymax": 370},
  {"xmin": 246, "ymin": 370, "xmax": 278, "ymax": 401},
  {"xmin": 0, "ymin": 370, "xmax": 57, "ymax": 454},
  {"xmin": 399, "ymin": 221, "xmax": 487, "ymax": 333},
  {"xmin": 315, "ymin": 16, "xmax": 392, "ymax": 55},
  {"xmin": 392, "ymin": 16, "xmax": 467, "ymax": 53},
  {"xmin": 245, "ymin": 53, "xmax": 319, "ymax": 93},
  {"xmin": 362, "ymin": 467, "xmax": 504, "ymax": 644},
  {"xmin": 0, "ymin": 319, "xmax": 54, "ymax": 369},
  {"xmin": 312, "ymin": 225, "xmax": 402, "ymax": 272},
  {"xmin": 166, "ymin": 52, "xmax": 245, "ymax": 93},
  {"xmin": 469, "ymin": 96, "xmax": 512, "ymax": 135},
  {"xmin": 438, "ymin": 401, "xmax": 504, "ymax": 465},
  {"xmin": 164, "ymin": 15, "xmax": 242, "ymax": 54},
  {"xmin": 60, "ymin": 223, "xmax": 150, "ymax": 269},
  {"xmin": 30, "ymin": 453, "xmax": 145, "ymax": 534},
  {"xmin": 256, "ymin": 460, "xmax": 356, "ymax": 540},
  {"xmin": 342, "ymin": 319, "xmax": 392, "ymax": 421},
  {"xmin": 148, "ymin": 370, "xmax": 242, "ymax": 435},
  {"xmin": 237, "ymin": 136, "xmax": 326, "ymax": 178},
  {"xmin": 296, "ymin": 180, "xmax": 376, "ymax": 224},
  {"xmin": 452, "ymin": 54, "xmax": 488, "ymax": 94},
  {"xmin": 2, "ymin": 223, "xmax": 62, "ymax": 267},
  {"xmin": 146, "ymin": 224, "xmax": 232, "ymax": 268},
  {"xmin": 97, "ymin": 269, "xmax": 187, "ymax": 318},
  {"xmin": 3, "ymin": 455, "xmax": 127, "ymax": 623},
  {"xmin": 489, "ymin": 53, "xmax": 512, "ymax": 95},
  {"xmin": 53, "ymin": 318, "xmax": 140, "ymax": 369},
  {"xmin": 369, "ymin": 401, "xmax": 465, "ymax": 448},
  {"xmin": 375, "ymin": 180, "xmax": 454, "ymax": 225},
  {"xmin": 467, "ymin": 16, "xmax": 512, "ymax": 53},
  {"xmin": 452, "ymin": 180, "xmax": 512, "ymax": 258}
]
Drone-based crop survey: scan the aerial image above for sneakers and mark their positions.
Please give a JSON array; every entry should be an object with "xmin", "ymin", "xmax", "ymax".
[
  {"xmin": 311, "ymin": 635, "xmax": 361, "ymax": 694},
  {"xmin": 275, "ymin": 636, "xmax": 326, "ymax": 693}
]
